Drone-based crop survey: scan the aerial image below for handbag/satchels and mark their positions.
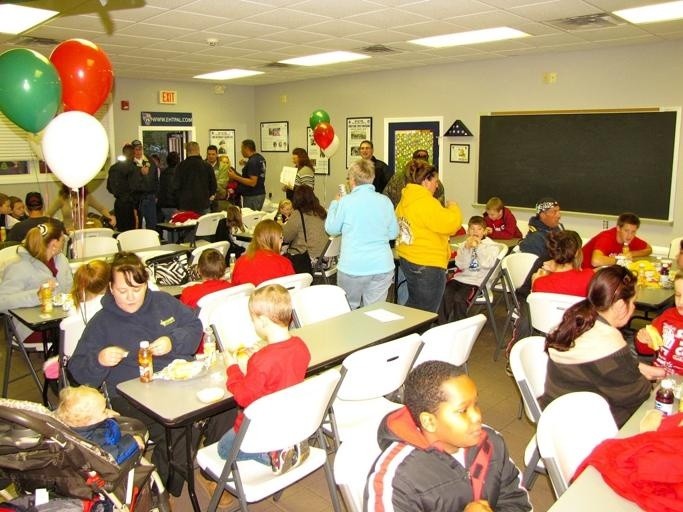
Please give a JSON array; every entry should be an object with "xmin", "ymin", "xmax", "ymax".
[
  {"xmin": 283, "ymin": 248, "xmax": 313, "ymax": 276},
  {"xmin": 147, "ymin": 251, "xmax": 190, "ymax": 287}
]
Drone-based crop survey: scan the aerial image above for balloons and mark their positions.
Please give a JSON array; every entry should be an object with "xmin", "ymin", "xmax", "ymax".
[
  {"xmin": 50, "ymin": 39, "xmax": 113, "ymax": 114},
  {"xmin": 41, "ymin": 110, "xmax": 110, "ymax": 193},
  {"xmin": 310, "ymin": 110, "xmax": 330, "ymax": 131},
  {"xmin": 313, "ymin": 122, "xmax": 334, "ymax": 150},
  {"xmin": 0, "ymin": 49, "xmax": 61, "ymax": 134}
]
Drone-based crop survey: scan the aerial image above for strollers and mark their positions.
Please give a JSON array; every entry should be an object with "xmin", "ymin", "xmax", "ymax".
[{"xmin": 0, "ymin": 398, "xmax": 177, "ymax": 512}]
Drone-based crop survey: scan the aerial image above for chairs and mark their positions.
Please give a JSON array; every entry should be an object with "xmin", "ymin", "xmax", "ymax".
[
  {"xmin": 2, "ymin": 212, "xmax": 488, "ymax": 508},
  {"xmin": 157, "ymin": 199, "xmax": 344, "ymax": 284},
  {"xmin": 509, "ymin": 336, "xmax": 681, "ymax": 508},
  {"xmin": 391, "ymin": 222, "xmax": 683, "ymax": 361}
]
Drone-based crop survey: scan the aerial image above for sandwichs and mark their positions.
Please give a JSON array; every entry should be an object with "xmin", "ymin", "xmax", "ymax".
[{"xmin": 646, "ymin": 324, "xmax": 664, "ymax": 351}]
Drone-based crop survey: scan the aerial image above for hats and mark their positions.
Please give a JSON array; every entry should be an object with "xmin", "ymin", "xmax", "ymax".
[
  {"xmin": 131, "ymin": 139, "xmax": 143, "ymax": 147},
  {"xmin": 25, "ymin": 191, "xmax": 42, "ymax": 208}
]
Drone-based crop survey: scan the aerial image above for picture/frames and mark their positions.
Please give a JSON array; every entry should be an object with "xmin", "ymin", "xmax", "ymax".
[
  {"xmin": 259, "ymin": 120, "xmax": 291, "ymax": 153},
  {"xmin": 449, "ymin": 142, "xmax": 469, "ymax": 164},
  {"xmin": 305, "ymin": 124, "xmax": 331, "ymax": 176},
  {"xmin": 207, "ymin": 128, "xmax": 236, "ymax": 170},
  {"xmin": 344, "ymin": 116, "xmax": 373, "ymax": 171}
]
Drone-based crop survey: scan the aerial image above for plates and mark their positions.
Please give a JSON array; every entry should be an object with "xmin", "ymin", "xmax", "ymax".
[{"xmin": 197, "ymin": 387, "xmax": 225, "ymax": 404}]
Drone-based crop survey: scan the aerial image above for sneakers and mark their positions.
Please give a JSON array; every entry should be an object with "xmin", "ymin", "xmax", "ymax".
[
  {"xmin": 291, "ymin": 440, "xmax": 310, "ymax": 470},
  {"xmin": 270, "ymin": 447, "xmax": 294, "ymax": 476},
  {"xmin": 196, "ymin": 470, "xmax": 234, "ymax": 510}
]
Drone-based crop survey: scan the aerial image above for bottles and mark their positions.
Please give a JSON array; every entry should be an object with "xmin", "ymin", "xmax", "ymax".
[
  {"xmin": 656, "ymin": 377, "xmax": 676, "ymax": 413},
  {"xmin": 40, "ymin": 283, "xmax": 53, "ymax": 312},
  {"xmin": 202, "ymin": 327, "xmax": 217, "ymax": 366},
  {"xmin": 621, "ymin": 243, "xmax": 630, "ymax": 256},
  {"xmin": 137, "ymin": 341, "xmax": 155, "ymax": 385},
  {"xmin": 637, "ymin": 254, "xmax": 669, "ymax": 287},
  {"xmin": 229, "ymin": 253, "xmax": 235, "ymax": 272}
]
